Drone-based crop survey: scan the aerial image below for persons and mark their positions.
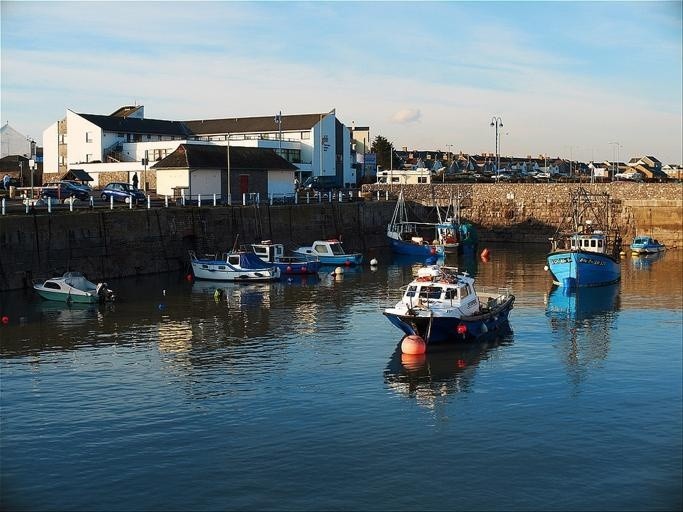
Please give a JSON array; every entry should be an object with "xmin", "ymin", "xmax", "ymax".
[
  {"xmin": 3, "ymin": 173, "xmax": 11, "ymax": 192},
  {"xmin": 293, "ymin": 176, "xmax": 299, "ymax": 191},
  {"xmin": 132, "ymin": 171, "xmax": 138, "ymax": 189}
]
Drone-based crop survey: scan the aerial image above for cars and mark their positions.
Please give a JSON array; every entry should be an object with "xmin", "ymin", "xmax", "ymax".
[
  {"xmin": 43, "ymin": 181, "xmax": 93, "ymax": 201},
  {"xmin": 102, "ymin": 182, "xmax": 147, "ymax": 206}
]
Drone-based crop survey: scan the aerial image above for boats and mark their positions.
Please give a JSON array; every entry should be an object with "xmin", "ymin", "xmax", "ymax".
[
  {"xmin": 382, "ymin": 244, "xmax": 516, "ymax": 355},
  {"xmin": 629, "ymin": 236, "xmax": 667, "ymax": 256},
  {"xmin": 385, "ymin": 184, "xmax": 474, "ymax": 256},
  {"xmin": 288, "ymin": 235, "xmax": 363, "ymax": 266},
  {"xmin": 185, "ymin": 235, "xmax": 281, "ymax": 281},
  {"xmin": 31, "ymin": 270, "xmax": 121, "ymax": 303},
  {"xmin": 249, "ymin": 240, "xmax": 319, "ymax": 274},
  {"xmin": 542, "ymin": 177, "xmax": 622, "ymax": 288}
]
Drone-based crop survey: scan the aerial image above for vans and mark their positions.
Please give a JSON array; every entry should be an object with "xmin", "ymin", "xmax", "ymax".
[{"xmin": 302, "ymin": 175, "xmax": 343, "ymax": 194}]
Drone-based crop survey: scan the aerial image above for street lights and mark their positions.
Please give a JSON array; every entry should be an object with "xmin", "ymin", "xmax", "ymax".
[
  {"xmin": 445, "ymin": 116, "xmax": 622, "ymax": 183},
  {"xmin": 275, "ymin": 111, "xmax": 287, "ymax": 158},
  {"xmin": 26, "ymin": 158, "xmax": 38, "ymax": 208},
  {"xmin": 223, "ymin": 131, "xmax": 238, "ymax": 209}
]
